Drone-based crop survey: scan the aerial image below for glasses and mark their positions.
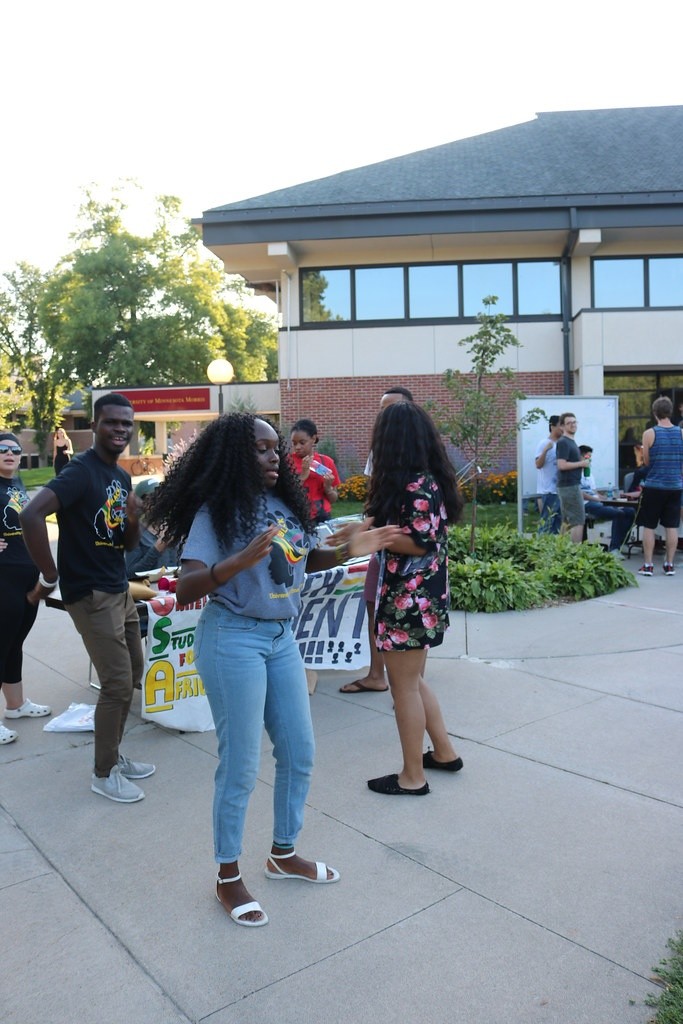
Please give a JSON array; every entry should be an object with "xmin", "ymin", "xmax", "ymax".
[{"xmin": 0, "ymin": 444, "xmax": 22, "ymax": 455}]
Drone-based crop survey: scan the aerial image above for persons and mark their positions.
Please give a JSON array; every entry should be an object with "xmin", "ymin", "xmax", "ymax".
[
  {"xmin": 124, "ymin": 479, "xmax": 186, "ymax": 579},
  {"xmin": 285, "ymin": 420, "xmax": 340, "ymax": 527},
  {"xmin": 325, "ymin": 388, "xmax": 466, "ymax": 795},
  {"xmin": 18, "ymin": 394, "xmax": 155, "ymax": 802},
  {"xmin": 0, "ymin": 432, "xmax": 51, "ymax": 745},
  {"xmin": 133, "ymin": 413, "xmax": 404, "ymax": 928},
  {"xmin": 535, "ymin": 397, "xmax": 683, "ymax": 578},
  {"xmin": 53, "ymin": 428, "xmax": 74, "ymax": 475}
]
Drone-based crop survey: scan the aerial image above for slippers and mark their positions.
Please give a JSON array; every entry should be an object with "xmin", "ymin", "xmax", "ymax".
[
  {"xmin": 340, "ymin": 679, "xmax": 389, "ymax": 693},
  {"xmin": 4, "ymin": 699, "xmax": 52, "ymax": 719},
  {"xmin": 0, "ymin": 719, "xmax": 19, "ymax": 745}
]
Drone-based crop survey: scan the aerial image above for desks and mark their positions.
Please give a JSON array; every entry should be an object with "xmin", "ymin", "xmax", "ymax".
[
  {"xmin": 45, "ymin": 561, "xmax": 369, "ymax": 735},
  {"xmin": 600, "ymin": 498, "xmax": 644, "ymax": 561}
]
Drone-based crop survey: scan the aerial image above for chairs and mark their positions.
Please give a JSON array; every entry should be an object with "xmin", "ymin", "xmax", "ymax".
[{"xmin": 624, "ymin": 472, "xmax": 634, "ymax": 493}]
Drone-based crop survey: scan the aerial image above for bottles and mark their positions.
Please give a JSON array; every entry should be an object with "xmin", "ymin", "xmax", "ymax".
[
  {"xmin": 308, "ymin": 460, "xmax": 332, "ymax": 479},
  {"xmin": 607, "ymin": 482, "xmax": 612, "ymax": 498},
  {"xmin": 583, "ymin": 456, "xmax": 590, "ymax": 477}
]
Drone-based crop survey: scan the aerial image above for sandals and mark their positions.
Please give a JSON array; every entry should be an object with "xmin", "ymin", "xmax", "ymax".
[
  {"xmin": 214, "ymin": 872, "xmax": 268, "ymax": 928},
  {"xmin": 265, "ymin": 850, "xmax": 340, "ymax": 884}
]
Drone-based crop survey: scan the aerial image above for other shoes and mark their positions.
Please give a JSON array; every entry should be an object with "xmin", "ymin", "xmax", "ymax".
[
  {"xmin": 423, "ymin": 749, "xmax": 464, "ymax": 772},
  {"xmin": 366, "ymin": 773, "xmax": 430, "ymax": 795}
]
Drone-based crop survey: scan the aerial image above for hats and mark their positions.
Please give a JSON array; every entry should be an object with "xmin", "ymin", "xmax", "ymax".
[{"xmin": 135, "ymin": 479, "xmax": 161, "ymax": 497}]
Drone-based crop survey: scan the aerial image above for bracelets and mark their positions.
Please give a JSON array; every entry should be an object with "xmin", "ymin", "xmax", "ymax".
[
  {"xmin": 209, "ymin": 562, "xmax": 227, "ymax": 586},
  {"xmin": 334, "ymin": 542, "xmax": 352, "ymax": 563},
  {"xmin": 38, "ymin": 572, "xmax": 60, "ymax": 588}
]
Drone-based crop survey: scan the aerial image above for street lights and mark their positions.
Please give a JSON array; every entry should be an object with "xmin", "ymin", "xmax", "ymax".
[{"xmin": 206, "ymin": 359, "xmax": 234, "ymax": 415}]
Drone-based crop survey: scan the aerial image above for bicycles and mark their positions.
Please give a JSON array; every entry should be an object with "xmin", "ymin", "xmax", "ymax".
[{"xmin": 131, "ymin": 452, "xmax": 156, "ymax": 476}]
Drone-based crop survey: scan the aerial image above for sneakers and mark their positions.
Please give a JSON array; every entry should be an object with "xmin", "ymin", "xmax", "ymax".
[
  {"xmin": 662, "ymin": 561, "xmax": 676, "ymax": 575},
  {"xmin": 610, "ymin": 549, "xmax": 625, "ymax": 561},
  {"xmin": 89, "ymin": 765, "xmax": 147, "ymax": 803},
  {"xmin": 114, "ymin": 754, "xmax": 157, "ymax": 779},
  {"xmin": 637, "ymin": 562, "xmax": 654, "ymax": 576}
]
